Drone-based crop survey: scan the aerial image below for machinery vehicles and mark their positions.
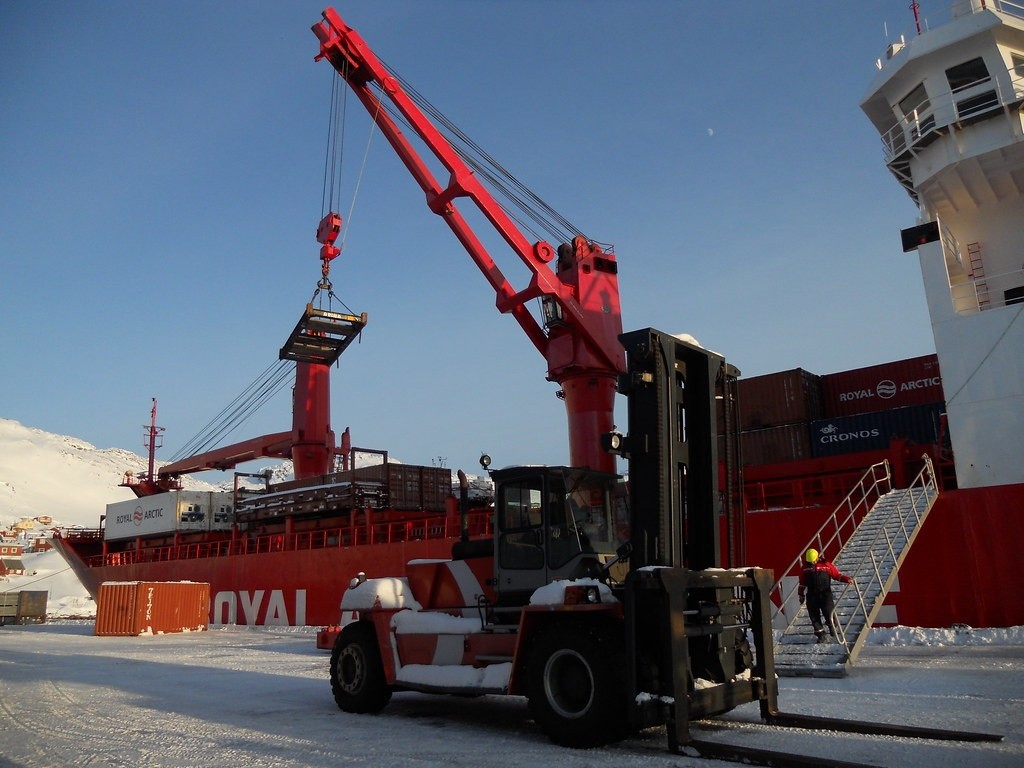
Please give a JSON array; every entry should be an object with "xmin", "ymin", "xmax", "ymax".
[{"xmin": 328, "ymin": 326, "xmax": 1007, "ymax": 767}]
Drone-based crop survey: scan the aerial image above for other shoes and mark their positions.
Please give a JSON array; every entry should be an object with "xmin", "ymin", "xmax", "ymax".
[
  {"xmin": 825, "ymin": 621, "xmax": 836, "ymax": 636},
  {"xmin": 814, "ymin": 627, "xmax": 826, "ymax": 643}
]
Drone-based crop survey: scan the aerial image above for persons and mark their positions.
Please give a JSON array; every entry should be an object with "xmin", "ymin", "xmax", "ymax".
[{"xmin": 796, "ymin": 547, "xmax": 855, "ymax": 643}]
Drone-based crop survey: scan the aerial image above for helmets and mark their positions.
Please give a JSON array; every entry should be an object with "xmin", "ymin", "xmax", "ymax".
[{"xmin": 806, "ymin": 549, "xmax": 818, "ymax": 562}]
